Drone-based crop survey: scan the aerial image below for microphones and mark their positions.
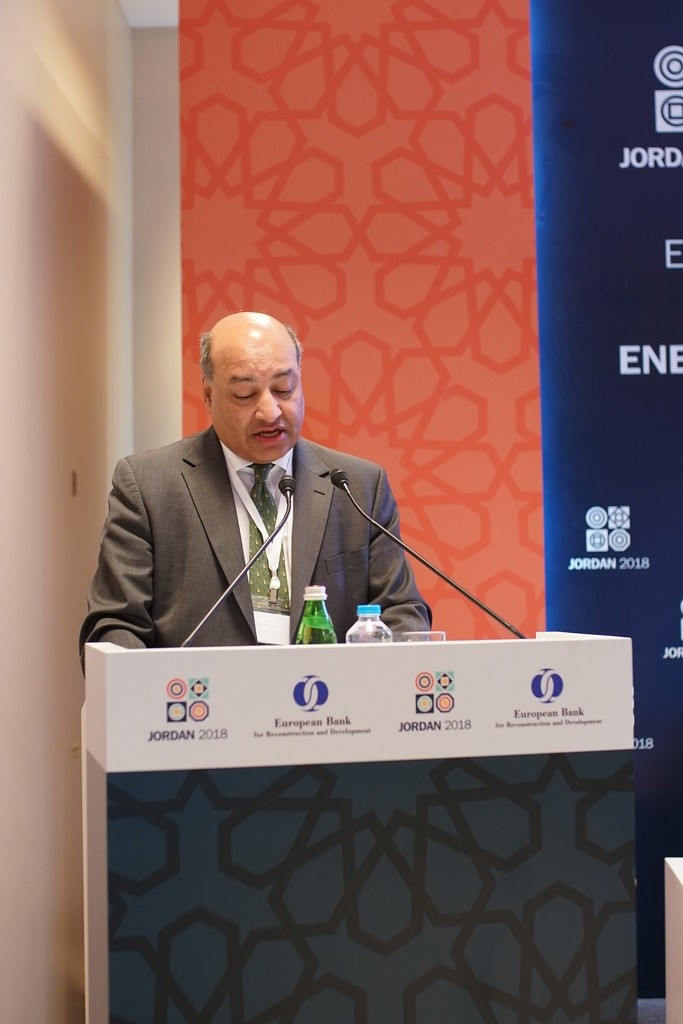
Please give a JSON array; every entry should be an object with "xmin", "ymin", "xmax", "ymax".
[
  {"xmin": 181, "ymin": 475, "xmax": 296, "ymax": 648},
  {"xmin": 330, "ymin": 468, "xmax": 525, "ymax": 638}
]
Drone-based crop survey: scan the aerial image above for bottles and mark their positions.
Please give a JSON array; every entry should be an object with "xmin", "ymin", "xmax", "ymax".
[
  {"xmin": 345, "ymin": 605, "xmax": 394, "ymax": 642},
  {"xmin": 295, "ymin": 585, "xmax": 337, "ymax": 645}
]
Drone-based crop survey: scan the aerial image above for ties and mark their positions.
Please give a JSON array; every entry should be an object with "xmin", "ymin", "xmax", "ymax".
[{"xmin": 247, "ymin": 462, "xmax": 291, "ymax": 615}]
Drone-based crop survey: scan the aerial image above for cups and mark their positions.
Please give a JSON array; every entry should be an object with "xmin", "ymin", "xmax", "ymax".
[{"xmin": 403, "ymin": 632, "xmax": 446, "ymax": 643}]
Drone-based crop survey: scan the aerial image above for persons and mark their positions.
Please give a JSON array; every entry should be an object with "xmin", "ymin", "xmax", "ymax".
[{"xmin": 78, "ymin": 312, "xmax": 432, "ymax": 678}]
